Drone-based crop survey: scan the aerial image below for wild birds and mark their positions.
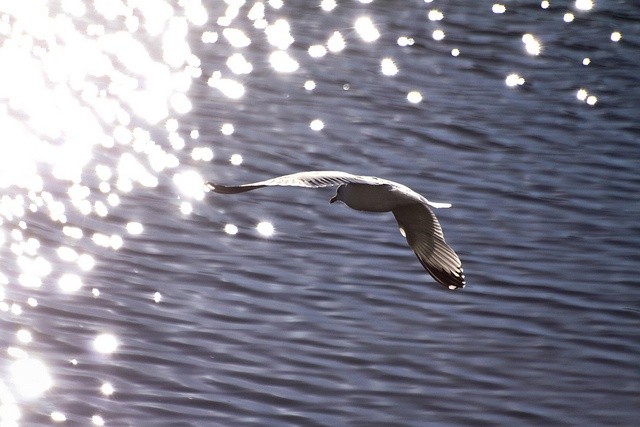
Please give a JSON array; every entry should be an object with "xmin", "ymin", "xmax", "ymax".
[{"xmin": 204, "ymin": 170, "xmax": 466, "ymax": 291}]
